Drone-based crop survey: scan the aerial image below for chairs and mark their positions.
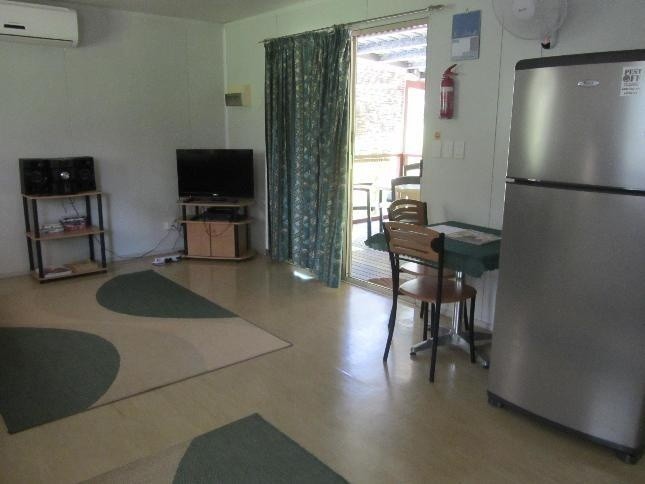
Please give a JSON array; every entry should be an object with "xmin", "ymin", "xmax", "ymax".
[
  {"xmin": 387, "ymin": 199, "xmax": 467, "ymax": 340},
  {"xmin": 352, "ymin": 163, "xmax": 420, "ymax": 239},
  {"xmin": 380, "ymin": 221, "xmax": 478, "ymax": 384}
]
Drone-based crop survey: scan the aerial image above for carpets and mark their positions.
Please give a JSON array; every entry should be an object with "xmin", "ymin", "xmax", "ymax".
[
  {"xmin": 0, "ymin": 259, "xmax": 292, "ymax": 436},
  {"xmin": 65, "ymin": 413, "xmax": 347, "ymax": 484}
]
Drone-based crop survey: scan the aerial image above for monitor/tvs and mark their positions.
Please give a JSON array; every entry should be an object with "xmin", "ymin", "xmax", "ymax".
[{"xmin": 176, "ymin": 148, "xmax": 255, "ymax": 202}]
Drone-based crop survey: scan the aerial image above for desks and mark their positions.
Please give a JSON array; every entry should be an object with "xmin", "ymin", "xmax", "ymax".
[{"xmin": 365, "ymin": 220, "xmax": 502, "ymax": 370}]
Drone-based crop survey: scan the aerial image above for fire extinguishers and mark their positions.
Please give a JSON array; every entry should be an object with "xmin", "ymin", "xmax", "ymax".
[{"xmin": 440, "ymin": 63, "xmax": 458, "ymax": 118}]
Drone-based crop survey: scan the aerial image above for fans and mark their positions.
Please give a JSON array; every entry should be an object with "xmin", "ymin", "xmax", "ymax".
[{"xmin": 492, "ymin": 0, "xmax": 569, "ymax": 49}]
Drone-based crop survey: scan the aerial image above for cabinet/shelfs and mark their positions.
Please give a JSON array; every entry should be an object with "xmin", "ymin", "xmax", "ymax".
[
  {"xmin": 176, "ymin": 197, "xmax": 257, "ymax": 263},
  {"xmin": 22, "ymin": 191, "xmax": 109, "ymax": 284}
]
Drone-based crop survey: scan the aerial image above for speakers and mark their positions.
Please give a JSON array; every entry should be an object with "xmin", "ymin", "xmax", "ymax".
[
  {"xmin": 71, "ymin": 155, "xmax": 97, "ymax": 193},
  {"xmin": 18, "ymin": 156, "xmax": 53, "ymax": 195}
]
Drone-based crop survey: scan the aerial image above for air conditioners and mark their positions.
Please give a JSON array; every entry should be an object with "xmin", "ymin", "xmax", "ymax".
[{"xmin": 0, "ymin": 0, "xmax": 79, "ymax": 48}]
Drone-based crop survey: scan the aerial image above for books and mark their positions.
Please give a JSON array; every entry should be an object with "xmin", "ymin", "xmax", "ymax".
[
  {"xmin": 39, "ymin": 216, "xmax": 87, "ymax": 235},
  {"xmin": 34, "ymin": 264, "xmax": 73, "ymax": 278},
  {"xmin": 445, "ymin": 228, "xmax": 502, "ymax": 246}
]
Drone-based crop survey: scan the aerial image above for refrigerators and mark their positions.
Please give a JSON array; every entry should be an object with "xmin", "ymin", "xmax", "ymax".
[{"xmin": 486, "ymin": 49, "xmax": 644, "ymax": 463}]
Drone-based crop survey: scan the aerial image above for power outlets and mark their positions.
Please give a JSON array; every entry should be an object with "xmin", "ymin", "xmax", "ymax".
[{"xmin": 164, "ymin": 221, "xmax": 177, "ymax": 230}]
